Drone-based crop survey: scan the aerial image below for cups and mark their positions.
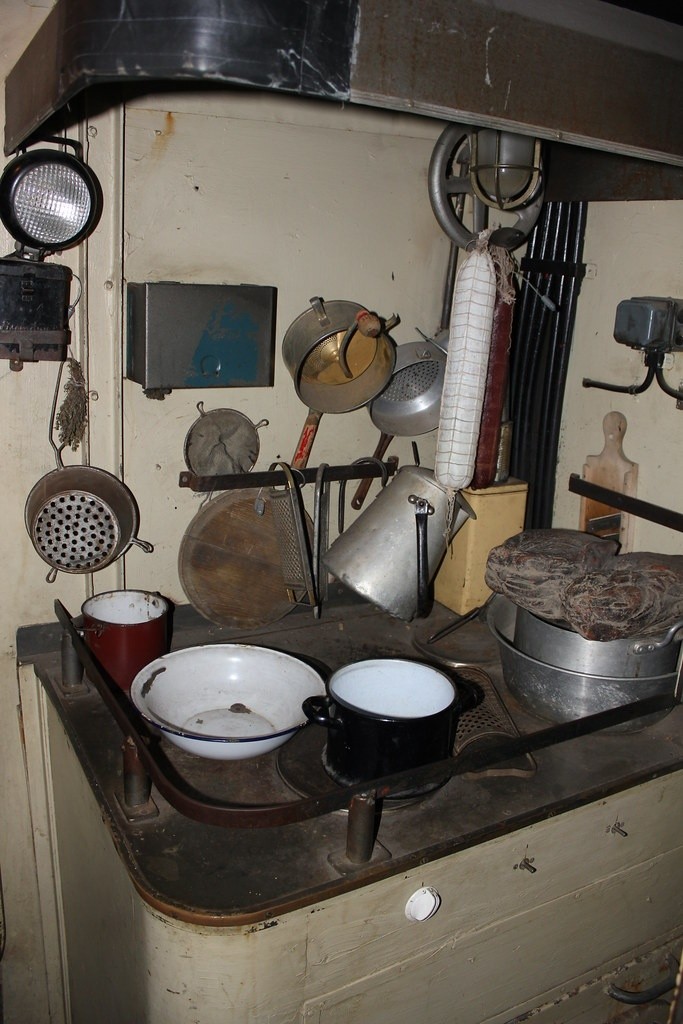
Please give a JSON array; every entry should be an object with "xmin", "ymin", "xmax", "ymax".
[{"xmin": 81, "ymin": 590, "xmax": 174, "ymax": 692}]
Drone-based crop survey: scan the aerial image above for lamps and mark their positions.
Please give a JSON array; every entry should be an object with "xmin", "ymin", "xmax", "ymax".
[
  {"xmin": 469, "ymin": 124, "xmax": 545, "ymax": 211},
  {"xmin": 0, "ymin": 133, "xmax": 104, "ymax": 262}
]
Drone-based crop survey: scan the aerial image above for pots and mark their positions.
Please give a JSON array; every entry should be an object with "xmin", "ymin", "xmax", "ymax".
[
  {"xmin": 284, "ymin": 297, "xmax": 397, "ymax": 470},
  {"xmin": 303, "ymin": 659, "xmax": 484, "ymax": 785},
  {"xmin": 481, "ymin": 586, "xmax": 683, "ymax": 737}
]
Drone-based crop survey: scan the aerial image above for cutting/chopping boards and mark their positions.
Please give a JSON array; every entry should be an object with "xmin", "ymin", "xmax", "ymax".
[{"xmin": 181, "ymin": 477, "xmax": 318, "ymax": 628}]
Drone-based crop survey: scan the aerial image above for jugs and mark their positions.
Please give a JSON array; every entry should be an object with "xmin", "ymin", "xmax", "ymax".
[{"xmin": 320, "ymin": 457, "xmax": 477, "ymax": 622}]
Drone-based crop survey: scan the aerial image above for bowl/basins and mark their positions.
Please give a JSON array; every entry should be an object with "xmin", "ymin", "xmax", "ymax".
[{"xmin": 131, "ymin": 644, "xmax": 326, "ymax": 759}]
